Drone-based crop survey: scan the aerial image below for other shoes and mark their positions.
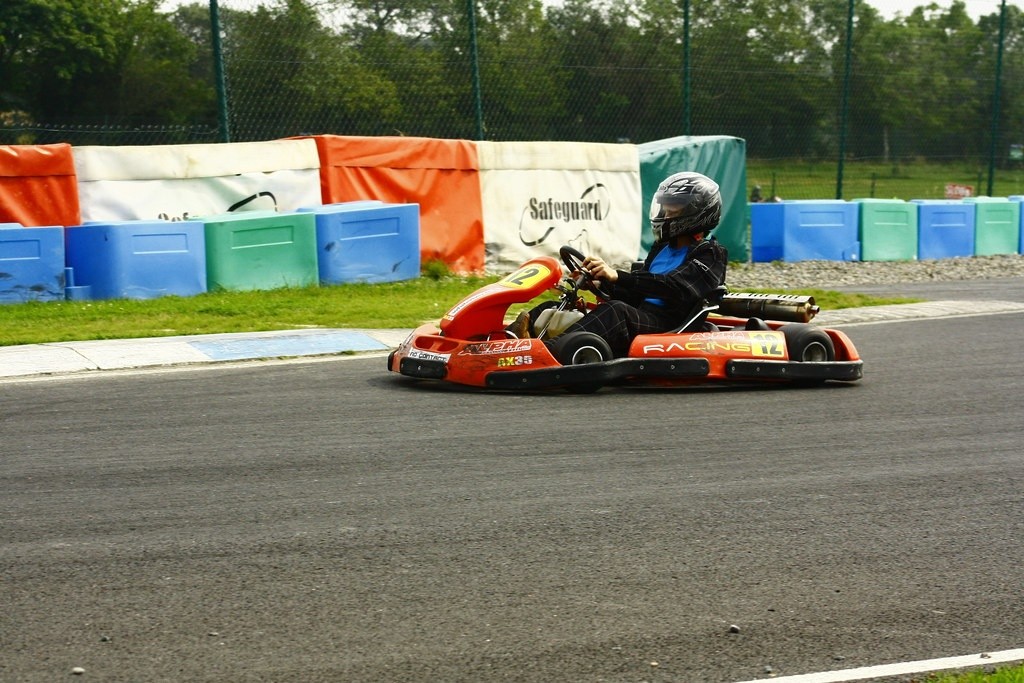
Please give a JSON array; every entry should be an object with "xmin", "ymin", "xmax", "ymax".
[{"xmin": 510, "ymin": 311, "xmax": 530, "ymax": 339}]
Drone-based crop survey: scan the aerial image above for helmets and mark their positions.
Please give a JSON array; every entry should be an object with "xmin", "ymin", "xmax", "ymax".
[{"xmin": 649, "ymin": 171, "xmax": 722, "ymax": 243}]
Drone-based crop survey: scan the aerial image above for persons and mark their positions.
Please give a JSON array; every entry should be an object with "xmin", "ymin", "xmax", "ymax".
[
  {"xmin": 750, "ymin": 186, "xmax": 762, "ymax": 202},
  {"xmin": 513, "ymin": 171, "xmax": 728, "ymax": 366}
]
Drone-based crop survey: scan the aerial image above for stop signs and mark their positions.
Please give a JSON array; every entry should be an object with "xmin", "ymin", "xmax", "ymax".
[{"xmin": 943, "ymin": 183, "xmax": 973, "ymax": 198}]
